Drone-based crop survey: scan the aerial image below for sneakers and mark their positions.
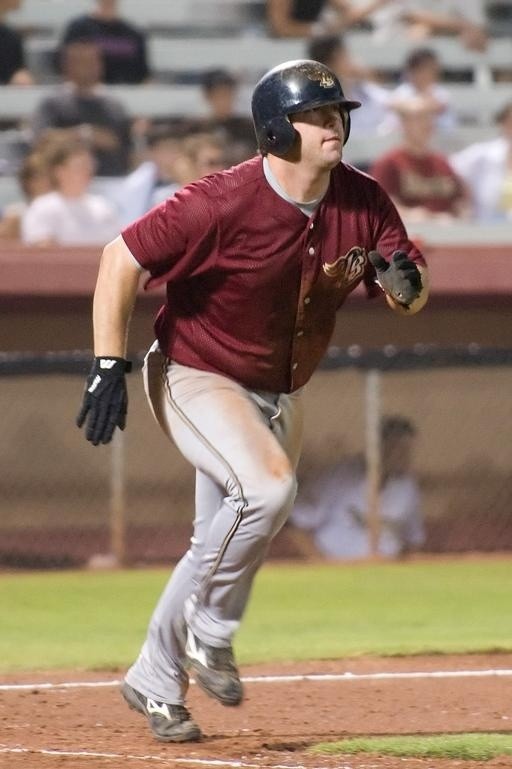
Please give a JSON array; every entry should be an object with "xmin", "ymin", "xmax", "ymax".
[
  {"xmin": 162, "ymin": 612, "xmax": 243, "ymax": 706},
  {"xmin": 120, "ymin": 682, "xmax": 201, "ymax": 743}
]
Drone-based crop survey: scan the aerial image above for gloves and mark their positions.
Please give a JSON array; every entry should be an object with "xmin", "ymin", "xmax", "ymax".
[
  {"xmin": 75, "ymin": 357, "xmax": 132, "ymax": 445},
  {"xmin": 367, "ymin": 249, "xmax": 423, "ymax": 307}
]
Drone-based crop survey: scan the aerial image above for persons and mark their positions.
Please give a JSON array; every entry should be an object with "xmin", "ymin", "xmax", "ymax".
[
  {"xmin": 285, "ymin": 412, "xmax": 426, "ymax": 561},
  {"xmin": 74, "ymin": 58, "xmax": 430, "ymax": 743}
]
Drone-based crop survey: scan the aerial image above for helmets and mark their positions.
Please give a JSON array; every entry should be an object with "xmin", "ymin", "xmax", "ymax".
[{"xmin": 251, "ymin": 59, "xmax": 363, "ymax": 158}]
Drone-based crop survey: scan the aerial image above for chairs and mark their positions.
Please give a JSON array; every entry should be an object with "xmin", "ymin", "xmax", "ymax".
[{"xmin": 2, "ymin": 0, "xmax": 510, "ymax": 247}]
